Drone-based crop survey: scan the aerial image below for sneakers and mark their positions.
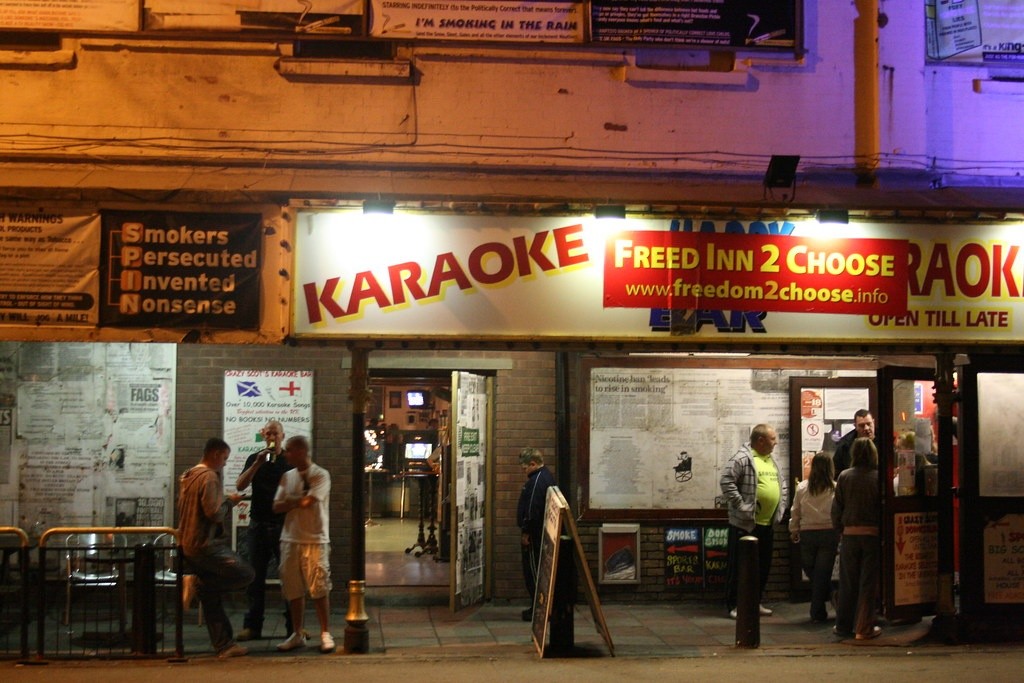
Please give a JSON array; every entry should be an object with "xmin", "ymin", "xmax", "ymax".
[
  {"xmin": 320, "ymin": 631, "xmax": 336, "ymax": 652},
  {"xmin": 276, "ymin": 631, "xmax": 306, "ymax": 650}
]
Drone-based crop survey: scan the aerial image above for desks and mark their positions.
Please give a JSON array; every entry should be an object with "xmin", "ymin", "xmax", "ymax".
[
  {"xmin": 363, "ymin": 468, "xmax": 389, "ymax": 527},
  {"xmin": 392, "ymin": 474, "xmax": 438, "ymax": 557}
]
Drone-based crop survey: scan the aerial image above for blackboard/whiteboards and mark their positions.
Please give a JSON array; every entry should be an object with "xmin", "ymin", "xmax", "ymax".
[
  {"xmin": 663, "ymin": 525, "xmax": 730, "ymax": 591},
  {"xmin": 530, "ymin": 487, "xmax": 616, "ymax": 660}
]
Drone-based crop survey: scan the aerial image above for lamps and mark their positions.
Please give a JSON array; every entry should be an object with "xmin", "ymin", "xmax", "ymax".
[
  {"xmin": 591, "ymin": 204, "xmax": 628, "ymax": 220},
  {"xmin": 362, "ymin": 199, "xmax": 396, "ymax": 215},
  {"xmin": 760, "ymin": 155, "xmax": 799, "ymax": 202},
  {"xmin": 813, "ymin": 208, "xmax": 850, "ymax": 225}
]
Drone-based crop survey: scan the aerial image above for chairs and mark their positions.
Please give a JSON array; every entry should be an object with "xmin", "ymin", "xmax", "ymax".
[
  {"xmin": 65, "ymin": 532, "xmax": 125, "ymax": 633},
  {"xmin": 152, "ymin": 532, "xmax": 204, "ymax": 628}
]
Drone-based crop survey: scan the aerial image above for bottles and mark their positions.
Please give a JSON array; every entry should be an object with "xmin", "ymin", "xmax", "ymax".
[{"xmin": 265, "ymin": 440, "xmax": 277, "ymax": 463}]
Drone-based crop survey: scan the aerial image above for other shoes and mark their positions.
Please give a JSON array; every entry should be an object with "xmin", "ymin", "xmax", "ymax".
[
  {"xmin": 856, "ymin": 626, "xmax": 881, "ymax": 640},
  {"xmin": 285, "ymin": 630, "xmax": 312, "ymax": 639},
  {"xmin": 219, "ymin": 643, "xmax": 248, "ymax": 659},
  {"xmin": 833, "ymin": 625, "xmax": 854, "ymax": 635},
  {"xmin": 731, "ymin": 608, "xmax": 736, "ymax": 617},
  {"xmin": 234, "ymin": 628, "xmax": 261, "ymax": 640},
  {"xmin": 760, "ymin": 605, "xmax": 773, "ymax": 614},
  {"xmin": 182, "ymin": 574, "xmax": 201, "ymax": 612}
]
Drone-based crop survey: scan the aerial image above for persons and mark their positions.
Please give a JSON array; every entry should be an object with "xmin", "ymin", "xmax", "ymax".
[
  {"xmin": 363, "ymin": 418, "xmax": 379, "ymax": 431},
  {"xmin": 233, "ymin": 418, "xmax": 311, "ymax": 641},
  {"xmin": 427, "ymin": 445, "xmax": 442, "ymax": 474},
  {"xmin": 789, "ymin": 451, "xmax": 839, "ymax": 625},
  {"xmin": 721, "ymin": 425, "xmax": 787, "ymax": 619},
  {"xmin": 426, "ymin": 418, "xmax": 439, "ymax": 432},
  {"xmin": 273, "ymin": 437, "xmax": 337, "ymax": 652},
  {"xmin": 834, "ymin": 410, "xmax": 938, "ymax": 615},
  {"xmin": 831, "ymin": 437, "xmax": 883, "ymax": 641},
  {"xmin": 517, "ymin": 448, "xmax": 557, "ymax": 620},
  {"xmin": 179, "ymin": 439, "xmax": 258, "ymax": 659}
]
